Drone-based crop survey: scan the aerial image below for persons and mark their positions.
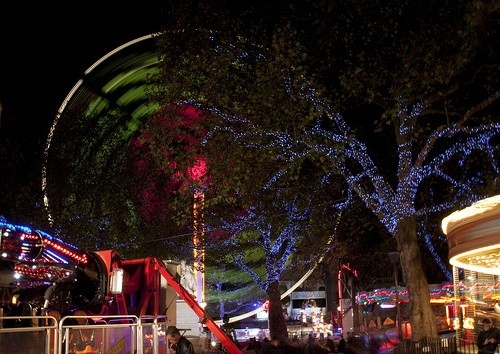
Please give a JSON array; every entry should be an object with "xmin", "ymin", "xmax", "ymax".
[
  {"xmin": 70, "ymin": 329, "xmax": 100, "ymax": 353},
  {"xmin": 474, "ymin": 317, "xmax": 500, "ymax": 354},
  {"xmin": 247, "ymin": 332, "xmax": 358, "ymax": 354},
  {"xmin": 165, "ymin": 325, "xmax": 195, "ymax": 354}
]
